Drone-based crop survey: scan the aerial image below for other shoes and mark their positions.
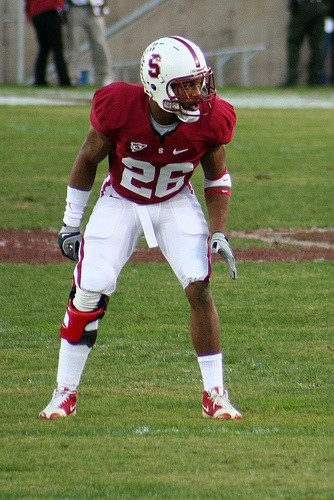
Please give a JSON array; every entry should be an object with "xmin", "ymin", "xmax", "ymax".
[
  {"xmin": 102, "ymin": 81, "xmax": 111, "ymax": 87},
  {"xmin": 71, "ymin": 79, "xmax": 78, "ymax": 85},
  {"xmin": 307, "ymin": 82, "xmax": 322, "ymax": 86},
  {"xmin": 280, "ymin": 83, "xmax": 296, "ymax": 88}
]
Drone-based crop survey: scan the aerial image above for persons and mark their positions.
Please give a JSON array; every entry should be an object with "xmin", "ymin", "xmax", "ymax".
[
  {"xmin": 38, "ymin": 35, "xmax": 244, "ymax": 420},
  {"xmin": 64, "ymin": 0, "xmax": 112, "ymax": 87},
  {"xmin": 25, "ymin": 0, "xmax": 71, "ymax": 88},
  {"xmin": 277, "ymin": 0, "xmax": 334, "ymax": 88}
]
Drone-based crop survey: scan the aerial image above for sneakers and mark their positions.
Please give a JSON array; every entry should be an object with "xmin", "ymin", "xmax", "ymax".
[
  {"xmin": 201, "ymin": 387, "xmax": 242, "ymax": 419},
  {"xmin": 38, "ymin": 388, "xmax": 77, "ymax": 420}
]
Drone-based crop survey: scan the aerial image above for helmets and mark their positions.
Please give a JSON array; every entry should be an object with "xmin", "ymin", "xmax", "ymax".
[{"xmin": 140, "ymin": 36, "xmax": 216, "ymax": 122}]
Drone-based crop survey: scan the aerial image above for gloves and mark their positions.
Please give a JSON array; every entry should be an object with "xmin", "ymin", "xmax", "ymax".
[
  {"xmin": 57, "ymin": 227, "xmax": 82, "ymax": 262},
  {"xmin": 209, "ymin": 232, "xmax": 237, "ymax": 280}
]
